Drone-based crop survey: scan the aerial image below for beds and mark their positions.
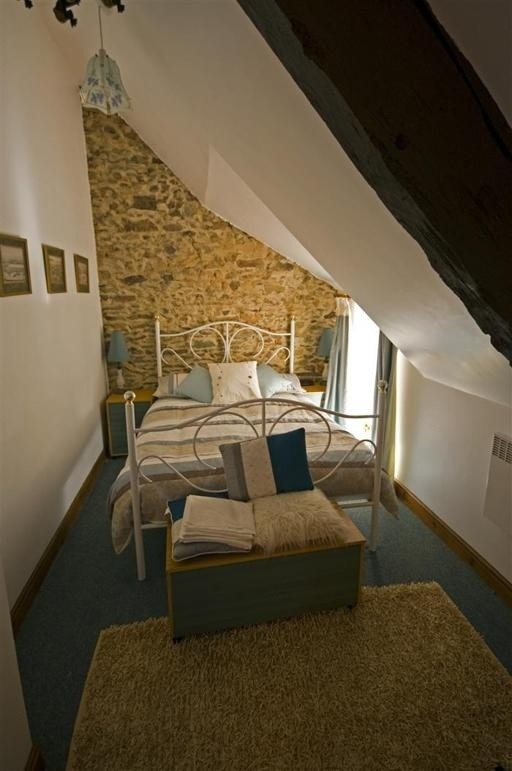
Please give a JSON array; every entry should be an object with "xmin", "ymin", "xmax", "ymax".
[{"xmin": 106, "ymin": 311, "xmax": 399, "ymax": 581}]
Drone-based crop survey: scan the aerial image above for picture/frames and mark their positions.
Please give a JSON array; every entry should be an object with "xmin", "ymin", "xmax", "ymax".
[
  {"xmin": 41, "ymin": 243, "xmax": 67, "ymax": 294},
  {"xmin": 73, "ymin": 254, "xmax": 90, "ymax": 293},
  {"xmin": 0, "ymin": 234, "xmax": 32, "ymax": 297}
]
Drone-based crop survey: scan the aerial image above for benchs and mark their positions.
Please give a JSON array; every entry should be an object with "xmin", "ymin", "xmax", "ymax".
[{"xmin": 163, "ymin": 496, "xmax": 366, "ymax": 641}]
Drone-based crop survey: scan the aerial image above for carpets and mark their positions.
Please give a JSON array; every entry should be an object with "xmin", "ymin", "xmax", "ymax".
[{"xmin": 70, "ymin": 578, "xmax": 510, "ymax": 769}]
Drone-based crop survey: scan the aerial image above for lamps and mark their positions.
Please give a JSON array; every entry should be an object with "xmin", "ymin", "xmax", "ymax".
[
  {"xmin": 317, "ymin": 326, "xmax": 335, "ymax": 386},
  {"xmin": 106, "ymin": 329, "xmax": 130, "ymax": 394},
  {"xmin": 23, "ymin": 0, "xmax": 132, "ymax": 115}
]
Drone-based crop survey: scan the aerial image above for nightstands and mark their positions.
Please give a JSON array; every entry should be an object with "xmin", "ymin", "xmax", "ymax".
[
  {"xmin": 105, "ymin": 387, "xmax": 156, "ymax": 458},
  {"xmin": 303, "ymin": 384, "xmax": 325, "ymax": 408}
]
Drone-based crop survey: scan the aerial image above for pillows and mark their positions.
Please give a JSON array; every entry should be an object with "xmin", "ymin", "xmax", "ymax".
[
  {"xmin": 153, "ymin": 372, "xmax": 191, "ymax": 400},
  {"xmin": 248, "ymin": 490, "xmax": 351, "ymax": 555},
  {"xmin": 207, "ymin": 360, "xmax": 263, "ymax": 406},
  {"xmin": 166, "ymin": 493, "xmax": 229, "ymax": 524},
  {"xmin": 279, "ymin": 372, "xmax": 306, "ymax": 395},
  {"xmin": 170, "ymin": 518, "xmax": 251, "ymax": 562},
  {"xmin": 217, "ymin": 428, "xmax": 314, "ymax": 502},
  {"xmin": 257, "ymin": 363, "xmax": 291, "ymax": 399},
  {"xmin": 175, "ymin": 367, "xmax": 213, "ymax": 403}
]
